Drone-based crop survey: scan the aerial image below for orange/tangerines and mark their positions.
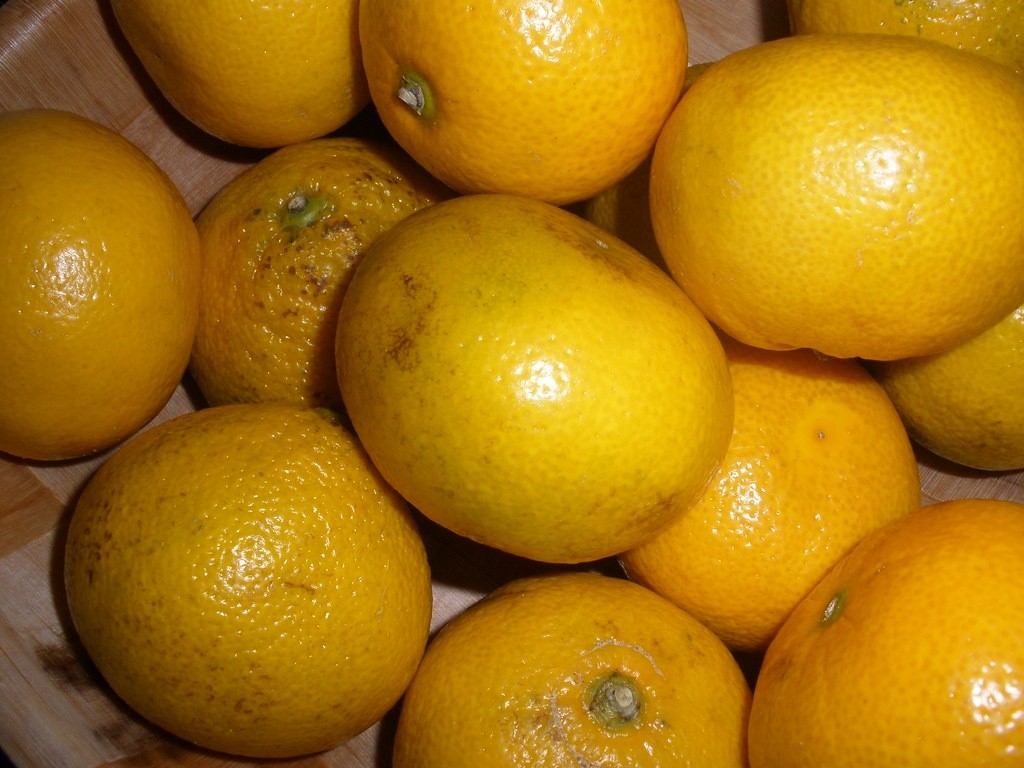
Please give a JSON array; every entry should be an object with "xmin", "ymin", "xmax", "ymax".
[{"xmin": 0, "ymin": 0, "xmax": 1024, "ymax": 768}]
